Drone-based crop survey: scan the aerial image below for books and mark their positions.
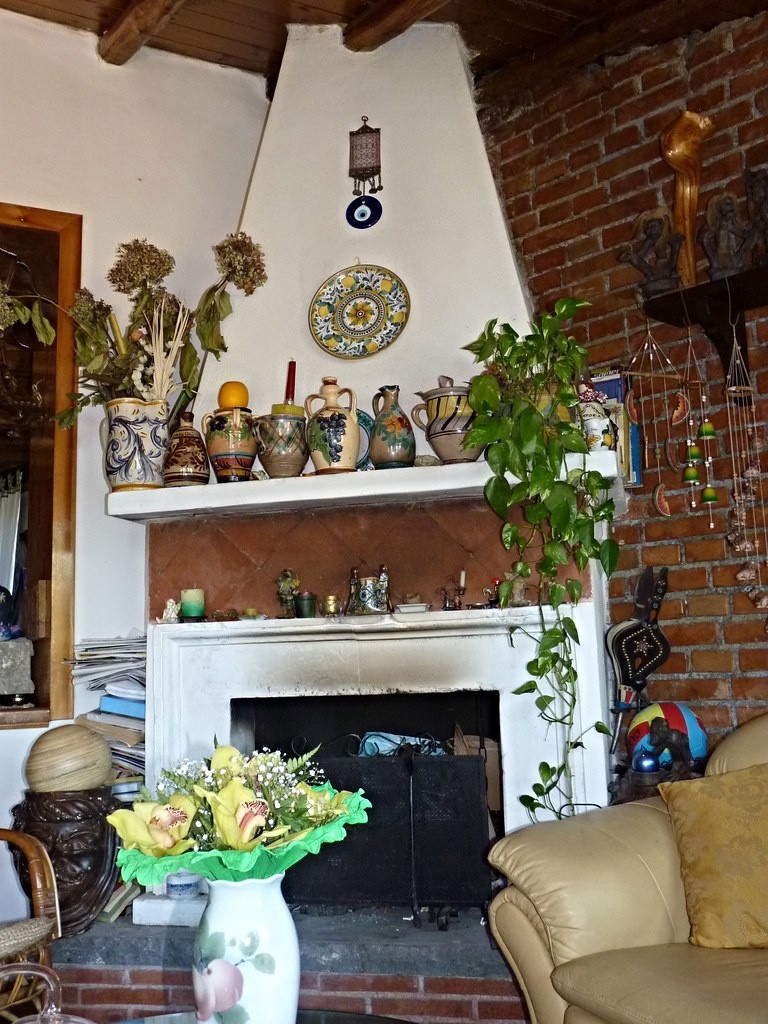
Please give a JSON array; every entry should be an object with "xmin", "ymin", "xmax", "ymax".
[
  {"xmin": 575, "ymin": 365, "xmax": 643, "ymax": 488},
  {"xmin": 60, "ymin": 638, "xmax": 147, "ymax": 802}
]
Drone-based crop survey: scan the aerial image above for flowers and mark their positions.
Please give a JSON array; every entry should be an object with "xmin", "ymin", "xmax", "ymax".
[
  {"xmin": 106, "ymin": 732, "xmax": 374, "ymax": 886},
  {"xmin": 0, "ymin": 232, "xmax": 269, "ymax": 426}
]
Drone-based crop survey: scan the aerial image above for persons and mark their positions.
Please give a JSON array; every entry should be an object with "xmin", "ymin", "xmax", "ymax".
[{"xmin": 8, "ymin": 797, "xmax": 141, "ymax": 938}]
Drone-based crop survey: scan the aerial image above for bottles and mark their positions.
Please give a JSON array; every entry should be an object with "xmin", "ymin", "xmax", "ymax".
[
  {"xmin": 369, "ymin": 384, "xmax": 416, "ymax": 470},
  {"xmin": 161, "ymin": 412, "xmax": 210, "ymax": 488},
  {"xmin": 344, "ymin": 406, "xmax": 375, "ymax": 471}
]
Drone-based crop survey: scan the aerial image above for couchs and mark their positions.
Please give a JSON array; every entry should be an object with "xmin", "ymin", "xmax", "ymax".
[{"xmin": 488, "ymin": 714, "xmax": 768, "ymax": 1024}]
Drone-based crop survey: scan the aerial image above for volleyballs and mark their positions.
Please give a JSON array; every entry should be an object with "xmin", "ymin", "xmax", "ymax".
[{"xmin": 628, "ymin": 702, "xmax": 709, "ymax": 770}]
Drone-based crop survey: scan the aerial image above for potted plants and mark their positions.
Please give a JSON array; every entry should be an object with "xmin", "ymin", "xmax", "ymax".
[{"xmin": 460, "ymin": 294, "xmax": 622, "ymax": 824}]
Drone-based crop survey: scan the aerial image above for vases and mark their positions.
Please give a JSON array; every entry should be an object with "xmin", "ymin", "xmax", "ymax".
[
  {"xmin": 193, "ymin": 870, "xmax": 301, "ymax": 1024},
  {"xmin": 100, "ymin": 397, "xmax": 170, "ymax": 491}
]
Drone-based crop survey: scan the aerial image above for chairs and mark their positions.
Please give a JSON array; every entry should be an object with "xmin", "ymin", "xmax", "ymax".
[{"xmin": 0, "ymin": 829, "xmax": 61, "ymax": 1021}]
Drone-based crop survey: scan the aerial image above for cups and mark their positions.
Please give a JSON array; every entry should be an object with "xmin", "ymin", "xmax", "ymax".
[
  {"xmin": 251, "ymin": 414, "xmax": 310, "ymax": 480},
  {"xmin": 297, "ymin": 594, "xmax": 318, "ymax": 618},
  {"xmin": 180, "ymin": 589, "xmax": 205, "ymax": 617}
]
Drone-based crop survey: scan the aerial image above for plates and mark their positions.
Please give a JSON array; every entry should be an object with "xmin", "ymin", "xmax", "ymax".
[{"xmin": 308, "ymin": 263, "xmax": 411, "ymax": 360}]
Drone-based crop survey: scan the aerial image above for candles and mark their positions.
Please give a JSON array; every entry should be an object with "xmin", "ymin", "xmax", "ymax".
[
  {"xmin": 460, "ymin": 567, "xmax": 466, "ymax": 587},
  {"xmin": 286, "ymin": 357, "xmax": 296, "ymax": 399}
]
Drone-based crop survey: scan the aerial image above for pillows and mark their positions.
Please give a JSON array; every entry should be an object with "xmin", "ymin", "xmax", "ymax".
[{"xmin": 658, "ymin": 761, "xmax": 768, "ymax": 950}]
